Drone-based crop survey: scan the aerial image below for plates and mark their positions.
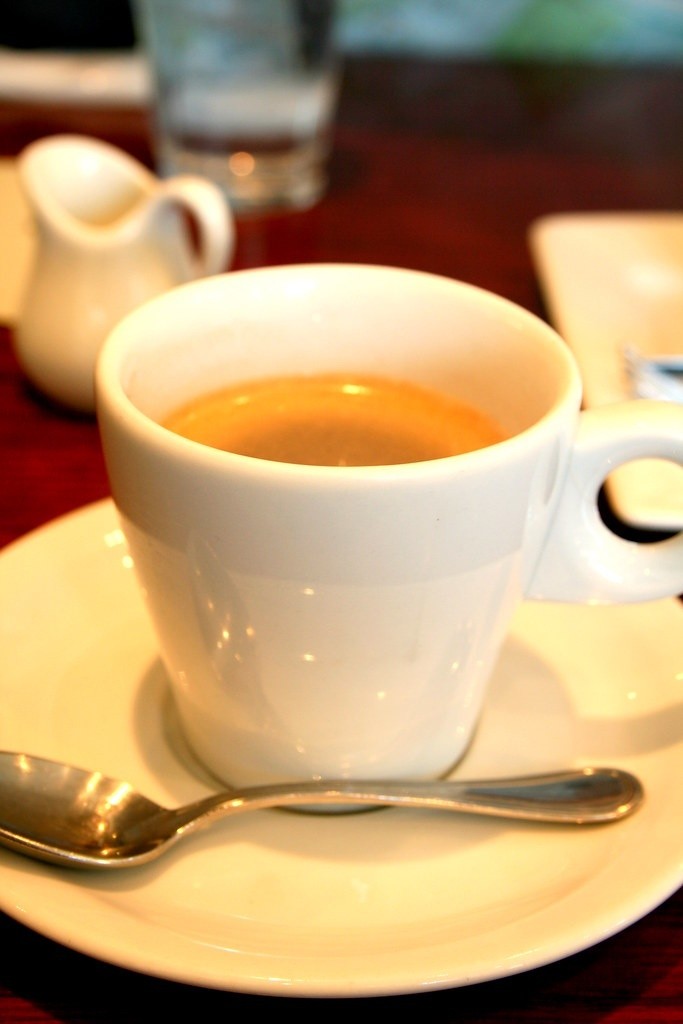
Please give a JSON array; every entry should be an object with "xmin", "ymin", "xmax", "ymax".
[
  {"xmin": 529, "ymin": 210, "xmax": 683, "ymax": 533},
  {"xmin": 1, "ymin": 483, "xmax": 683, "ymax": 1001}
]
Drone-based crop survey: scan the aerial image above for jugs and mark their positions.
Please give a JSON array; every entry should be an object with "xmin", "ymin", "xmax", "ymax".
[{"xmin": 13, "ymin": 135, "xmax": 234, "ymax": 416}]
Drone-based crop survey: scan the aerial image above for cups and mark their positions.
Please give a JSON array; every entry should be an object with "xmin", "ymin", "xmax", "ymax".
[
  {"xmin": 96, "ymin": 263, "xmax": 683, "ymax": 807},
  {"xmin": 133, "ymin": 0, "xmax": 344, "ymax": 211}
]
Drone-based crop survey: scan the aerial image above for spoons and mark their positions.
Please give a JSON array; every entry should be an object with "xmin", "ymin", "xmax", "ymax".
[{"xmin": 0, "ymin": 749, "xmax": 643, "ymax": 870}]
